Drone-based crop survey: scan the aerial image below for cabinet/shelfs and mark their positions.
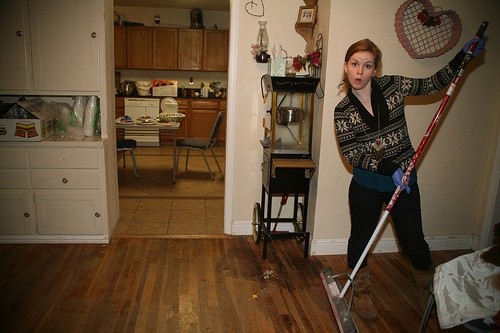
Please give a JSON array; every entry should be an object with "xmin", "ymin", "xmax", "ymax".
[
  {"xmin": 0, "ymin": 0, "xmax": 106, "ymax": 235},
  {"xmin": 116, "ymin": 98, "xmax": 125, "ymax": 141},
  {"xmin": 114, "ymin": 26, "xmax": 227, "ymax": 71},
  {"xmin": 253, "ymin": 76, "xmax": 319, "ymax": 261},
  {"xmin": 160, "ymin": 99, "xmax": 226, "ymax": 144}
]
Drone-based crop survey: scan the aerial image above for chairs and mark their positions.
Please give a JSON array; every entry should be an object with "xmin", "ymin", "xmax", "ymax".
[
  {"xmin": 117, "ymin": 139, "xmax": 139, "ymax": 179},
  {"xmin": 170, "ymin": 110, "xmax": 224, "ymax": 184}
]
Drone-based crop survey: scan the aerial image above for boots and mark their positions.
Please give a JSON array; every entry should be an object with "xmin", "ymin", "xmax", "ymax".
[
  {"xmin": 348, "ymin": 264, "xmax": 379, "ymax": 320},
  {"xmin": 410, "ymin": 262, "xmax": 438, "ymax": 317}
]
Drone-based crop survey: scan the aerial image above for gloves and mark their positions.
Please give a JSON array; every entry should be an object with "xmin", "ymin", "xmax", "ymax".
[
  {"xmin": 463, "ymin": 38, "xmax": 484, "ymax": 55},
  {"xmin": 392, "ymin": 168, "xmax": 411, "ymax": 194}
]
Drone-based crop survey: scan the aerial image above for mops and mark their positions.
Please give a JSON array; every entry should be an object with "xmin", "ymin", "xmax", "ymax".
[{"xmin": 320, "ymin": 20, "xmax": 489, "ymax": 333}]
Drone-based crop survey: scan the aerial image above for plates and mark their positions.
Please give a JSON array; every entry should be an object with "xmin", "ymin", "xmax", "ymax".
[
  {"xmin": 138, "ymin": 122, "xmax": 155, "ymax": 125},
  {"xmin": 117, "ymin": 123, "xmax": 135, "ymax": 125}
]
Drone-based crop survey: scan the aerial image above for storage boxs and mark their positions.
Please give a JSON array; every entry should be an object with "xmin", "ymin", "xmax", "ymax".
[{"xmin": 0, "ymin": 98, "xmax": 54, "ymax": 141}]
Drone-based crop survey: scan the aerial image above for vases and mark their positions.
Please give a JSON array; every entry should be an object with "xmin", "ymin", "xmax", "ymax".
[{"xmin": 295, "ymin": 62, "xmax": 310, "ymax": 75}]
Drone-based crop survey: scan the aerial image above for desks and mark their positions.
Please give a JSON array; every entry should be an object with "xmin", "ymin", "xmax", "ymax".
[{"xmin": 115, "ymin": 122, "xmax": 180, "ymax": 185}]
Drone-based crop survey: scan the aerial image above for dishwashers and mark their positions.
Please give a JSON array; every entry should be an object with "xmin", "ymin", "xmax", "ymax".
[{"xmin": 124, "ymin": 98, "xmax": 159, "ymax": 146}]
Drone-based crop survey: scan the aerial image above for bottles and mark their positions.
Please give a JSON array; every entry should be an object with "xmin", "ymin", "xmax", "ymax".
[
  {"xmin": 114, "ymin": 10, "xmax": 120, "ymax": 25},
  {"xmin": 257, "ymin": 21, "xmax": 269, "ymax": 47}
]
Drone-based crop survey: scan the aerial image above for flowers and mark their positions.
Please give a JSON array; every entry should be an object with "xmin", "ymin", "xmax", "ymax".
[{"xmin": 292, "ymin": 51, "xmax": 322, "ymax": 72}]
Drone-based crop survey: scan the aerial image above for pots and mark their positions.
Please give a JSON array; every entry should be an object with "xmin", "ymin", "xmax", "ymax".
[
  {"xmin": 266, "ymin": 107, "xmax": 301, "ymax": 122},
  {"xmin": 182, "ymin": 83, "xmax": 204, "ymax": 99},
  {"xmin": 120, "ymin": 81, "xmax": 135, "ymax": 96}
]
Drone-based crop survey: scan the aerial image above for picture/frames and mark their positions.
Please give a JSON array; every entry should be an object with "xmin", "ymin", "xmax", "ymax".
[{"xmin": 296, "ymin": 6, "xmax": 318, "ymax": 23}]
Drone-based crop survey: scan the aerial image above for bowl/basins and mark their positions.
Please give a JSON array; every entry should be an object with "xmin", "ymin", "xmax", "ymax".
[{"xmin": 162, "ymin": 113, "xmax": 185, "ymax": 122}]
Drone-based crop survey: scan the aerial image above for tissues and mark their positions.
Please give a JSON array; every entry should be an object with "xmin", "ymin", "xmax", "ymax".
[{"xmin": 268, "ymin": 43, "xmax": 287, "ymax": 77}]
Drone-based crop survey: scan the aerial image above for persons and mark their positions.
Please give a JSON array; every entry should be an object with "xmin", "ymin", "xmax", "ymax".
[{"xmin": 332, "ymin": 33, "xmax": 487, "ymax": 319}]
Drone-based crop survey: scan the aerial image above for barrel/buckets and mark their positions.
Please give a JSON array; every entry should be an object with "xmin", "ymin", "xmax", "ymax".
[{"xmin": 135, "ymin": 81, "xmax": 151, "ymax": 96}]
